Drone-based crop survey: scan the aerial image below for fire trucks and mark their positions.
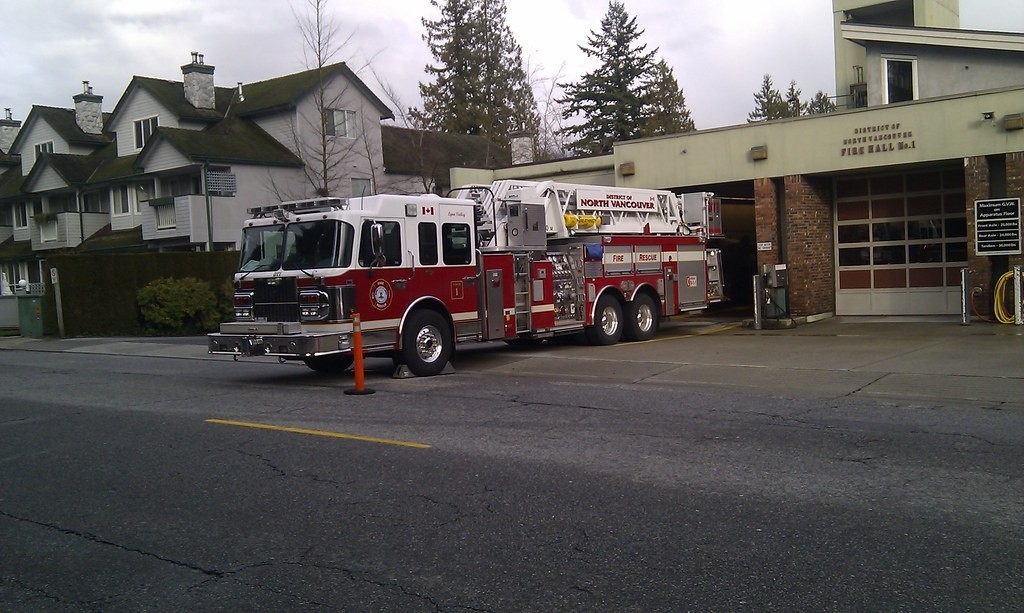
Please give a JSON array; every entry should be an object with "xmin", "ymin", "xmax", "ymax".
[{"xmin": 208, "ymin": 178, "xmax": 727, "ymax": 378}]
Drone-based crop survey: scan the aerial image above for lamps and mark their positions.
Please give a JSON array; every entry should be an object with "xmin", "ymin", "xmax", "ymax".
[
  {"xmin": 751, "ymin": 145, "xmax": 768, "ymax": 160},
  {"xmin": 620, "ymin": 161, "xmax": 635, "ymax": 176},
  {"xmin": 1004, "ymin": 112, "xmax": 1024, "ymax": 130}
]
